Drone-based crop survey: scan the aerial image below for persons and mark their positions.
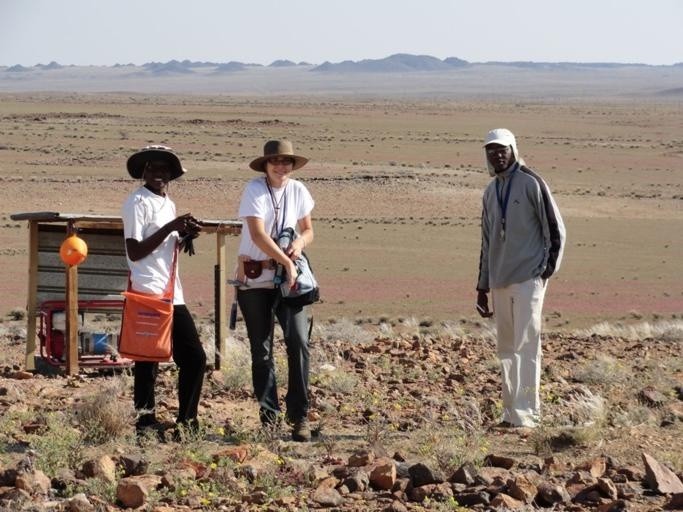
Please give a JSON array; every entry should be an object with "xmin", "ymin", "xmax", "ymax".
[
  {"xmin": 121, "ymin": 142, "xmax": 207, "ymax": 437},
  {"xmin": 475, "ymin": 128, "xmax": 567, "ymax": 428},
  {"xmin": 236, "ymin": 140, "xmax": 320, "ymax": 441}
]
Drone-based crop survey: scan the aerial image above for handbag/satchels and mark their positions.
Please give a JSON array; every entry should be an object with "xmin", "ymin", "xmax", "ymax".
[
  {"xmin": 119, "ymin": 291, "xmax": 174, "ymax": 362},
  {"xmin": 276, "ymin": 228, "xmax": 320, "ymax": 307}
]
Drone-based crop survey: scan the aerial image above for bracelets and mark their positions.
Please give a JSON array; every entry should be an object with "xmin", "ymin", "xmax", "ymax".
[{"xmin": 298, "ymin": 235, "xmax": 307, "ymax": 248}]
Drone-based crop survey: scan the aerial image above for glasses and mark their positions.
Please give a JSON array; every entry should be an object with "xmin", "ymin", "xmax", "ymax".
[
  {"xmin": 487, "ymin": 149, "xmax": 507, "ymax": 157},
  {"xmin": 146, "ymin": 165, "xmax": 171, "ymax": 174},
  {"xmin": 267, "ymin": 157, "xmax": 293, "ymax": 165}
]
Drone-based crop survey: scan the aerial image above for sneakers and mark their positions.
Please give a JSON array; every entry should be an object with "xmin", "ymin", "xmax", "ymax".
[
  {"xmin": 292, "ymin": 417, "xmax": 312, "ymax": 442},
  {"xmin": 136, "ymin": 419, "xmax": 199, "ymax": 442}
]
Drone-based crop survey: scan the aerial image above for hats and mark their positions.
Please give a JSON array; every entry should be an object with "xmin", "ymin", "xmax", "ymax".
[
  {"xmin": 127, "ymin": 145, "xmax": 187, "ymax": 180},
  {"xmin": 249, "ymin": 140, "xmax": 309, "ymax": 172},
  {"xmin": 481, "ymin": 128, "xmax": 525, "ymax": 177}
]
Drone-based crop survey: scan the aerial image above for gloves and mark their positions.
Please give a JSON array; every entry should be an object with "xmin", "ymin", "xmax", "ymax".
[{"xmin": 177, "ymin": 231, "xmax": 199, "ymax": 256}]
